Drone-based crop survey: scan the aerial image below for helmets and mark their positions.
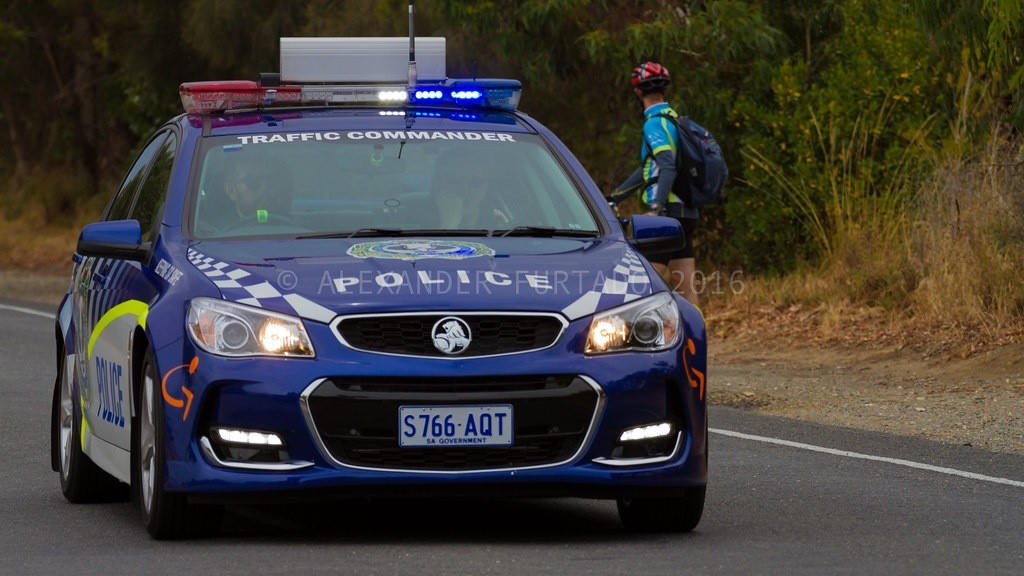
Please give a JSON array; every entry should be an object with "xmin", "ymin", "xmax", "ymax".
[{"xmin": 630, "ymin": 62, "xmax": 670, "ymax": 87}]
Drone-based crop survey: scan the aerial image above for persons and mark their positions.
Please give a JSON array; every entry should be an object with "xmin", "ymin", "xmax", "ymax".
[
  {"xmin": 605, "ymin": 62, "xmax": 698, "ymax": 311},
  {"xmin": 199, "ymin": 148, "xmax": 508, "ymax": 235}
]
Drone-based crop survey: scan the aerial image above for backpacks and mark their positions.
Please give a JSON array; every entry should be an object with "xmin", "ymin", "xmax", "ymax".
[{"xmin": 643, "ymin": 114, "xmax": 726, "ymax": 208}]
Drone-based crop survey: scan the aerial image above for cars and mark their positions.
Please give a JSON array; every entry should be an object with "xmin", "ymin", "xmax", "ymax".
[{"xmin": 47, "ymin": 28, "xmax": 710, "ymax": 533}]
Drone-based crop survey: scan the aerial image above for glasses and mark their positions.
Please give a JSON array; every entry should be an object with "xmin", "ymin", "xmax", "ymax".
[{"xmin": 233, "ymin": 173, "xmax": 279, "ymax": 190}]
